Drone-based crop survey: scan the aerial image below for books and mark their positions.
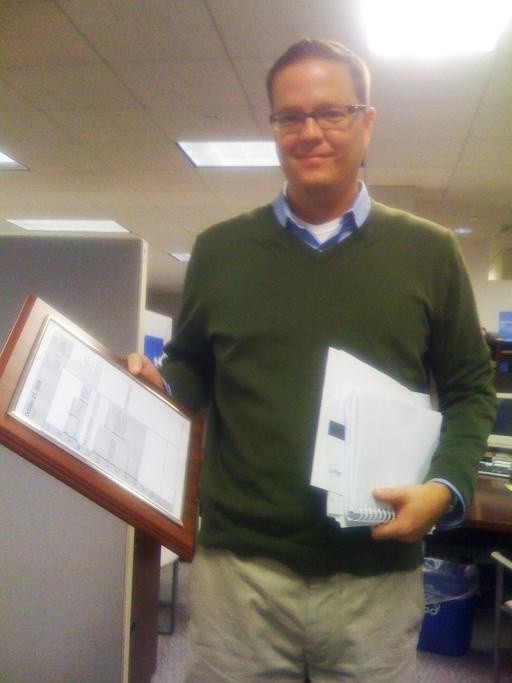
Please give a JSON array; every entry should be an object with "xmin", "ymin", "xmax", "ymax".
[{"xmin": 310, "ymin": 348, "xmax": 443, "ymax": 531}]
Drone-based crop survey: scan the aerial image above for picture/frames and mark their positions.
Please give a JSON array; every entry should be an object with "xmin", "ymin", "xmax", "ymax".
[{"xmin": 1, "ymin": 294, "xmax": 203, "ymax": 564}]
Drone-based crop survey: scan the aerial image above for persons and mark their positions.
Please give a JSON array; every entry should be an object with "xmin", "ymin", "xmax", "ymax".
[{"xmin": 104, "ymin": 33, "xmax": 501, "ymax": 683}]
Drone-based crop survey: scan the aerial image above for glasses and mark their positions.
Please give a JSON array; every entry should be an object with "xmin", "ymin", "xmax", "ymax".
[{"xmin": 268, "ymin": 101, "xmax": 369, "ymax": 136}]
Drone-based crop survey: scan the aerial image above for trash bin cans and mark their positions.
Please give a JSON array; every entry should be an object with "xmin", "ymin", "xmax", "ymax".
[{"xmin": 417, "ymin": 556, "xmax": 486, "ymax": 656}]
